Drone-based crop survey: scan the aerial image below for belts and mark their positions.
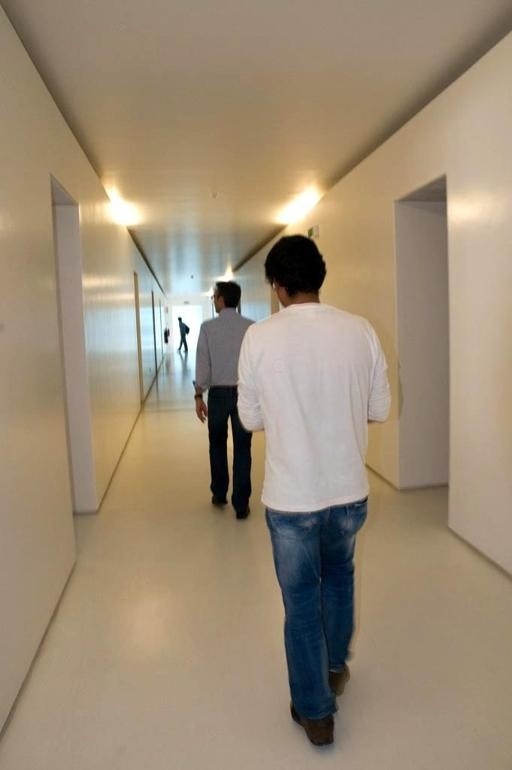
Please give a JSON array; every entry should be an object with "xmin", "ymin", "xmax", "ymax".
[{"xmin": 210, "ymin": 385, "xmax": 239, "ymax": 391}]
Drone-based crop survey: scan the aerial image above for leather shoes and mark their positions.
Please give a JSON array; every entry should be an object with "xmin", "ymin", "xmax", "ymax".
[
  {"xmin": 234, "ymin": 506, "xmax": 251, "ymax": 521},
  {"xmin": 210, "ymin": 493, "xmax": 229, "ymax": 506},
  {"xmin": 289, "ymin": 695, "xmax": 336, "ymax": 747},
  {"xmin": 327, "ymin": 660, "xmax": 352, "ymax": 698}
]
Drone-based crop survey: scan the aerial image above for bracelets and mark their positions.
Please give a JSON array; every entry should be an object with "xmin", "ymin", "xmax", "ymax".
[{"xmin": 194, "ymin": 394, "xmax": 204, "ymax": 398}]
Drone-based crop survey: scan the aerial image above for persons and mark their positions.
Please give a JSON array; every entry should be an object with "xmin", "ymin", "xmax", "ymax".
[
  {"xmin": 193, "ymin": 280, "xmax": 259, "ymax": 519},
  {"xmin": 177, "ymin": 316, "xmax": 190, "ymax": 352},
  {"xmin": 237, "ymin": 233, "xmax": 391, "ymax": 747}
]
